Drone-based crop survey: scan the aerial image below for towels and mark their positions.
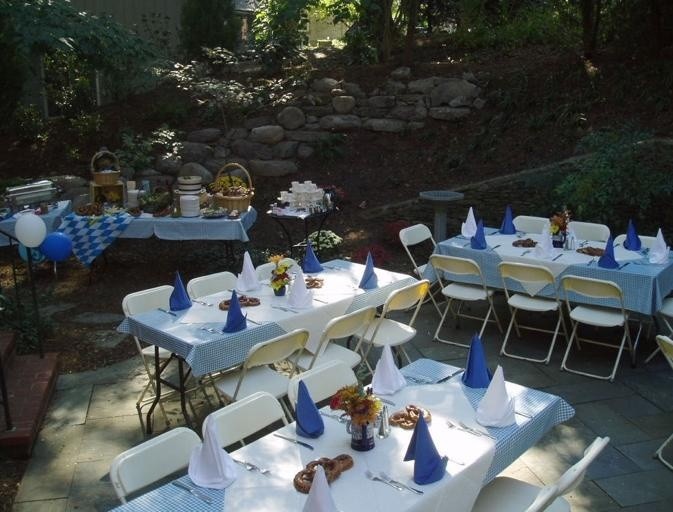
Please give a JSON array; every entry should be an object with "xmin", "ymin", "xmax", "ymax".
[
  {"xmin": 299, "ymin": 461, "xmax": 338, "ymax": 511},
  {"xmin": 473, "ymin": 364, "xmax": 517, "ymax": 430},
  {"xmin": 401, "ymin": 409, "xmax": 449, "ymax": 485},
  {"xmin": 290, "ymin": 376, "xmax": 328, "ymax": 439},
  {"xmin": 188, "ymin": 411, "xmax": 239, "ymax": 493}
]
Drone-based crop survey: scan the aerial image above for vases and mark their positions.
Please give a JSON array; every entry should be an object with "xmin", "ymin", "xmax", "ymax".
[{"xmin": 342, "ymin": 419, "xmax": 379, "ymax": 452}]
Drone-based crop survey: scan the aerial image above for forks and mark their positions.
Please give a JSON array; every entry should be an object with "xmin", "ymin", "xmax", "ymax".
[
  {"xmin": 191, "ymin": 298, "xmax": 214, "ymax": 307},
  {"xmin": 446, "ymin": 420, "xmax": 481, "ymax": 437},
  {"xmin": 460, "ymin": 422, "xmax": 497, "ymax": 440},
  {"xmin": 364, "ymin": 468, "xmax": 403, "ymax": 492},
  {"xmin": 195, "ymin": 324, "xmax": 224, "ymax": 335},
  {"xmin": 228, "ymin": 452, "xmax": 271, "ymax": 474},
  {"xmin": 379, "ymin": 470, "xmax": 424, "ymax": 495}
]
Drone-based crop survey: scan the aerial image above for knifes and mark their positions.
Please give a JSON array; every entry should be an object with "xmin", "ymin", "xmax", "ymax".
[
  {"xmin": 371, "ymin": 395, "xmax": 396, "ymax": 407},
  {"xmin": 157, "ymin": 307, "xmax": 177, "ymax": 317},
  {"xmin": 244, "ymin": 317, "xmax": 260, "ymax": 325},
  {"xmin": 272, "ymin": 433, "xmax": 315, "ymax": 452},
  {"xmin": 312, "ymin": 298, "xmax": 328, "ymax": 304},
  {"xmin": 270, "ymin": 306, "xmax": 297, "ymax": 313},
  {"xmin": 227, "ymin": 288, "xmax": 242, "ymax": 295},
  {"xmin": 171, "ymin": 479, "xmax": 213, "ymax": 504},
  {"xmin": 463, "ymin": 231, "xmax": 630, "ymax": 270}
]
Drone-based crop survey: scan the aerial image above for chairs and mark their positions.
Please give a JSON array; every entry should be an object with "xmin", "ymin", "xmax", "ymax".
[
  {"xmin": 201, "ymin": 390, "xmax": 290, "ymax": 448},
  {"xmin": 0, "ymin": 149, "xmax": 672, "ymax": 425},
  {"xmin": 396, "ymin": 213, "xmax": 671, "ymax": 384},
  {"xmin": 115, "ymin": 256, "xmax": 433, "ymax": 419},
  {"xmin": 470, "ymin": 434, "xmax": 613, "ymax": 511},
  {"xmin": 649, "ymin": 333, "xmax": 671, "ymax": 474},
  {"xmin": 107, "ymin": 424, "xmax": 206, "ymax": 507}
]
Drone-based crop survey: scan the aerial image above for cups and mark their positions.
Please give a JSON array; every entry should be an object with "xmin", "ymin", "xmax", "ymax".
[
  {"xmin": 125, "ymin": 180, "xmax": 137, "ymax": 208},
  {"xmin": 39, "ymin": 202, "xmax": 48, "ymax": 214},
  {"xmin": 273, "ymin": 181, "xmax": 325, "ymax": 215}
]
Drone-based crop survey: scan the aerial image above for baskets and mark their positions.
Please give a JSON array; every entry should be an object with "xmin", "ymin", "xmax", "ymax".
[
  {"xmin": 91, "ymin": 151, "xmax": 120, "ymax": 186},
  {"xmin": 213, "ymin": 162, "xmax": 252, "ymax": 212}
]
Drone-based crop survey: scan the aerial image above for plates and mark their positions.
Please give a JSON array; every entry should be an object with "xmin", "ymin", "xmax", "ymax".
[
  {"xmin": 203, "ymin": 211, "xmax": 223, "ymax": 218},
  {"xmin": 180, "ymin": 197, "xmax": 201, "ymax": 218}
]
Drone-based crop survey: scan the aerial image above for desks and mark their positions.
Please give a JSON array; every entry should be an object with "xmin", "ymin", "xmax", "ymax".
[
  {"xmin": 265, "ymin": 200, "xmax": 332, "ymax": 261},
  {"xmin": 1, "ymin": 196, "xmax": 261, "ymax": 280}
]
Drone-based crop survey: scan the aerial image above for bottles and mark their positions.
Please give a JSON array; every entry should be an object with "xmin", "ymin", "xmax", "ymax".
[
  {"xmin": 375, "ymin": 404, "xmax": 391, "ymax": 438},
  {"xmin": 171, "ymin": 199, "xmax": 180, "ymax": 217}
]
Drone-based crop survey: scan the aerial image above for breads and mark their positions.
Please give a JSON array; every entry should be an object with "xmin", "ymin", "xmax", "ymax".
[
  {"xmin": 294, "ymin": 454, "xmax": 354, "ymax": 495},
  {"xmin": 219, "ymin": 295, "xmax": 260, "ymax": 310},
  {"xmin": 389, "ymin": 404, "xmax": 432, "ymax": 430}
]
